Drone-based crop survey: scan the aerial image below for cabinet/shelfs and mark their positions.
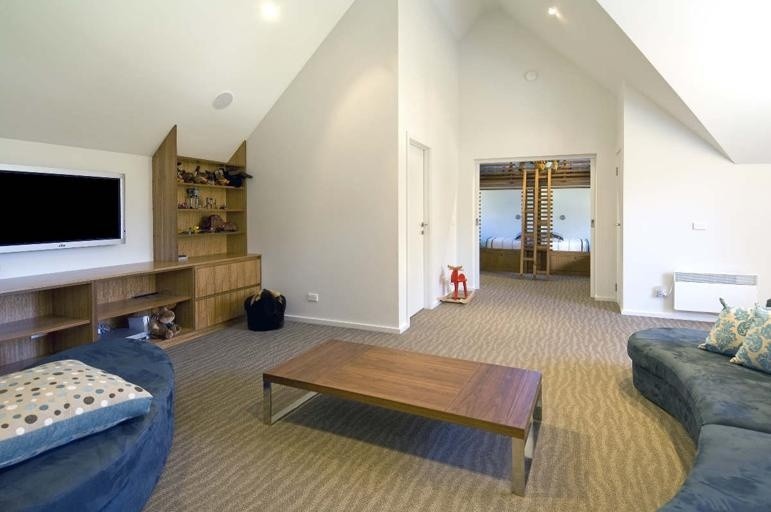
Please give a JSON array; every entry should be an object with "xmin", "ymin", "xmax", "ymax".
[
  {"xmin": 0, "ymin": 252, "xmax": 263, "ymax": 378},
  {"xmin": 154, "ymin": 123, "xmax": 248, "ymax": 261}
]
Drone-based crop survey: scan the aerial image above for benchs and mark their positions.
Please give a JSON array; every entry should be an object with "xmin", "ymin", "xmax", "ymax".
[{"xmin": 0, "ymin": 334, "xmax": 177, "ymax": 512}]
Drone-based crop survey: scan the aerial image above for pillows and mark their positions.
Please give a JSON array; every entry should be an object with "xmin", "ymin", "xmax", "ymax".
[
  {"xmin": 0, "ymin": 357, "xmax": 152, "ymax": 467},
  {"xmin": 697, "ymin": 299, "xmax": 771, "ymax": 375}
]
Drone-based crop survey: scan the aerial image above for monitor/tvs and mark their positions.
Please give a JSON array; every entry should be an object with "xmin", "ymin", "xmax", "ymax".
[{"xmin": 0, "ymin": 165, "xmax": 127, "ymax": 252}]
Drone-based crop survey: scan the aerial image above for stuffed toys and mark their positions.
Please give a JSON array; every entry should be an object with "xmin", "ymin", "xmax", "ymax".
[{"xmin": 149, "ymin": 307, "xmax": 182, "ymax": 339}]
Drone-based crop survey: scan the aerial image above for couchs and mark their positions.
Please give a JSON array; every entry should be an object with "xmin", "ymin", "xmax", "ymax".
[{"xmin": 625, "ymin": 292, "xmax": 771, "ymax": 512}]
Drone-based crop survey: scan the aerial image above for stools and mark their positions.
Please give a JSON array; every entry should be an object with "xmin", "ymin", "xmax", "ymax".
[{"xmin": 243, "ymin": 288, "xmax": 287, "ymax": 332}]
